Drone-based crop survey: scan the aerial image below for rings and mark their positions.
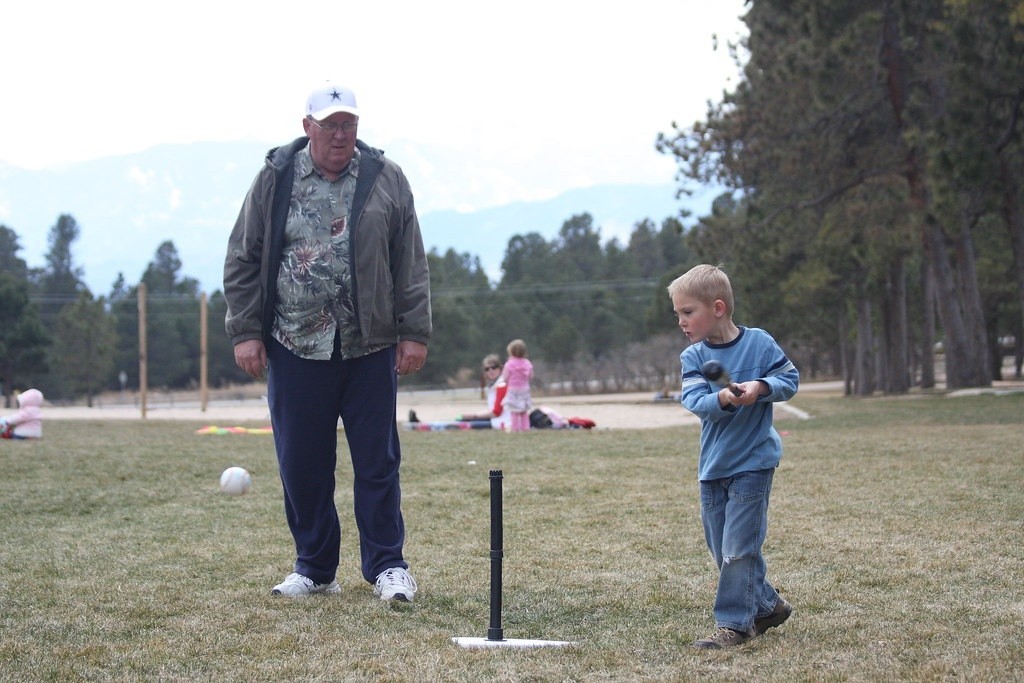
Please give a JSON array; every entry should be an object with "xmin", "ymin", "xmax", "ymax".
[{"xmin": 416, "ymin": 368, "xmax": 420, "ymax": 370}]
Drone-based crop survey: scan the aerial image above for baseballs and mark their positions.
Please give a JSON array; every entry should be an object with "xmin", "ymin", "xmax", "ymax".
[{"xmin": 219, "ymin": 467, "xmax": 253, "ymax": 497}]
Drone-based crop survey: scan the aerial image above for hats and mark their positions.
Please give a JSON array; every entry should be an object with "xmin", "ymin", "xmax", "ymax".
[{"xmin": 306, "ymin": 87, "xmax": 360, "ymax": 121}]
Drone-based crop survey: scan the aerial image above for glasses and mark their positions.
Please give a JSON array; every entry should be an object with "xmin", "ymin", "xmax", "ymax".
[
  {"xmin": 484, "ymin": 365, "xmax": 498, "ymax": 371},
  {"xmin": 311, "ymin": 119, "xmax": 359, "ymax": 134}
]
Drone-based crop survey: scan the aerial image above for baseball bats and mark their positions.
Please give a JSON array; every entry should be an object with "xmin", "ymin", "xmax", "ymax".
[{"xmin": 702, "ymin": 360, "xmax": 742, "ymax": 398}]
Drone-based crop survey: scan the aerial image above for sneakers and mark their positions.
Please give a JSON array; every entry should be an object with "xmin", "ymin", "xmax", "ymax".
[
  {"xmin": 754, "ymin": 596, "xmax": 793, "ymax": 635},
  {"xmin": 693, "ymin": 627, "xmax": 757, "ymax": 649},
  {"xmin": 271, "ymin": 573, "xmax": 341, "ymax": 596},
  {"xmin": 373, "ymin": 567, "xmax": 417, "ymax": 602}
]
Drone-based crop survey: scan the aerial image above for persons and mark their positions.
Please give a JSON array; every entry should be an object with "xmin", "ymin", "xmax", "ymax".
[
  {"xmin": 408, "ymin": 410, "xmax": 420, "ymax": 431},
  {"xmin": 667, "ymin": 265, "xmax": 799, "ymax": 648},
  {"xmin": 0, "ymin": 388, "xmax": 45, "ymax": 439},
  {"xmin": 472, "ymin": 354, "xmax": 513, "ymax": 432},
  {"xmin": 502, "ymin": 339, "xmax": 534, "ymax": 433},
  {"xmin": 224, "ymin": 82, "xmax": 433, "ymax": 602}
]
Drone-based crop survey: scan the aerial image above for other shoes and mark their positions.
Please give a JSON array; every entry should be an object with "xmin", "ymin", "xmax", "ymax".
[{"xmin": 409, "ymin": 409, "xmax": 420, "ymax": 429}]
